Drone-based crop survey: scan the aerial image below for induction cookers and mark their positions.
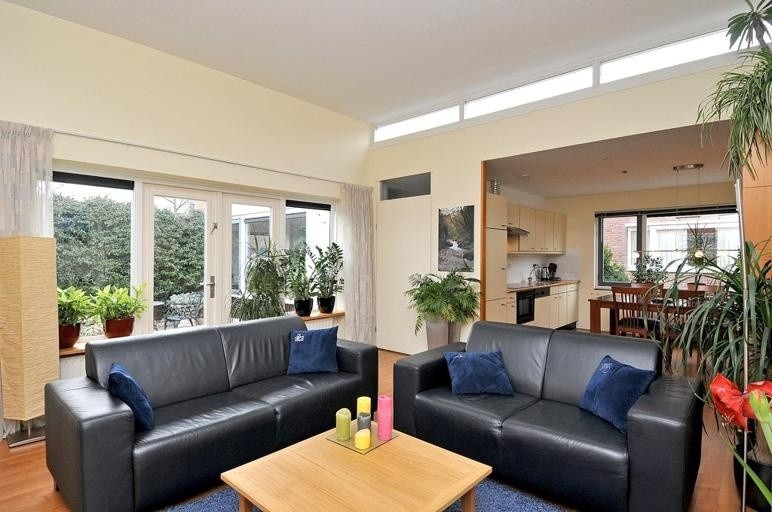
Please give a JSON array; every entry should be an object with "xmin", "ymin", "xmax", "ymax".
[{"xmin": 507, "ymin": 282, "xmax": 535, "ymax": 289}]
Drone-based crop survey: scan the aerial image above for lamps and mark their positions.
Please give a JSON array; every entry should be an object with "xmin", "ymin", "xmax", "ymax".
[{"xmin": 0, "ymin": 235, "xmax": 62, "ymax": 448}]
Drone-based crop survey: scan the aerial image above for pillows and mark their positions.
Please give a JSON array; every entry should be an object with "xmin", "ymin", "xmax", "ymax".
[
  {"xmin": 286, "ymin": 324, "xmax": 341, "ymax": 375},
  {"xmin": 442, "ymin": 348, "xmax": 515, "ymax": 399},
  {"xmin": 577, "ymin": 355, "xmax": 657, "ymax": 434},
  {"xmin": 107, "ymin": 362, "xmax": 155, "ymax": 431}
]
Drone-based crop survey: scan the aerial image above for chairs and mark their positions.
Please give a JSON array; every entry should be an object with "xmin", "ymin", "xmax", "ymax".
[
  {"xmin": 630, "ymin": 282, "xmax": 664, "ymax": 299},
  {"xmin": 164, "ymin": 290, "xmax": 204, "ymax": 330},
  {"xmin": 610, "ymin": 286, "xmax": 660, "ymax": 340},
  {"xmin": 662, "ymin": 289, "xmax": 707, "ymax": 371},
  {"xmin": 686, "ymin": 282, "xmax": 729, "ymax": 360}
]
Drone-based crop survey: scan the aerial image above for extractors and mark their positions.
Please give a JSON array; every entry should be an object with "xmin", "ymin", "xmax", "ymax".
[{"xmin": 507, "ymin": 226, "xmax": 529, "ymax": 236}]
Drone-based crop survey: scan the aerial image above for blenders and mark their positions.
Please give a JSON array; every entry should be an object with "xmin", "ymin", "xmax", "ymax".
[{"xmin": 549, "ymin": 263, "xmax": 561, "ymax": 281}]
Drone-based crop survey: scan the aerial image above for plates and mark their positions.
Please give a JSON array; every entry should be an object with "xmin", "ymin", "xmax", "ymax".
[{"xmin": 651, "ymin": 298, "xmax": 674, "ymax": 303}]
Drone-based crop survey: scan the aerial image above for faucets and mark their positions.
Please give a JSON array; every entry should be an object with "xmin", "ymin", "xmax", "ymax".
[{"xmin": 527, "ymin": 266, "xmax": 541, "ymax": 285}]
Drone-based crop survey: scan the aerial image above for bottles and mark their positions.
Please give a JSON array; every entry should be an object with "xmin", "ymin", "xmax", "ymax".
[{"xmin": 336, "ymin": 394, "xmax": 392, "ymax": 449}]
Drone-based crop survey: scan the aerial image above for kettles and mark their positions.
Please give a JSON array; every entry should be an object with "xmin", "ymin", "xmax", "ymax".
[{"xmin": 541, "ymin": 267, "xmax": 550, "ymax": 281}]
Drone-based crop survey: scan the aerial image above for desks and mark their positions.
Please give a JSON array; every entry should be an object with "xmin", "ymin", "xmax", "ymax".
[
  {"xmin": 588, "ymin": 293, "xmax": 739, "ymax": 344},
  {"xmin": 153, "ymin": 300, "xmax": 164, "ymax": 331}
]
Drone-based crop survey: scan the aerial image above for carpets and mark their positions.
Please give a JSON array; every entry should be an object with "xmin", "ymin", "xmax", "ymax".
[{"xmin": 162, "ymin": 470, "xmax": 570, "ymax": 512}]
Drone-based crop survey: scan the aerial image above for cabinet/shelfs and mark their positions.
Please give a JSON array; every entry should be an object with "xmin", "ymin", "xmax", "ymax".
[
  {"xmin": 508, "ymin": 293, "xmax": 517, "ymax": 324},
  {"xmin": 567, "ymin": 283, "xmax": 580, "ymax": 327},
  {"xmin": 487, "ymin": 298, "xmax": 507, "ymax": 326},
  {"xmin": 551, "ymin": 284, "xmax": 567, "ymax": 333},
  {"xmin": 534, "ymin": 296, "xmax": 551, "ymax": 330},
  {"xmin": 485, "ymin": 192, "xmax": 507, "ymax": 301}
]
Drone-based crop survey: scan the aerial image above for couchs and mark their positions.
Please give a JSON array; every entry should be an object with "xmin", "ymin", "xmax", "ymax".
[
  {"xmin": 392, "ymin": 315, "xmax": 707, "ymax": 512},
  {"xmin": 42, "ymin": 313, "xmax": 379, "ymax": 512}
]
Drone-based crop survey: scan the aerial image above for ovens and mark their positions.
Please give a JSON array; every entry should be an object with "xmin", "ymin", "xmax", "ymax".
[{"xmin": 516, "ymin": 290, "xmax": 534, "ymax": 325}]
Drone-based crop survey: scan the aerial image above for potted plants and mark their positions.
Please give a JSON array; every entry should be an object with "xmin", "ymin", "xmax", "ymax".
[
  {"xmin": 640, "ymin": 229, "xmax": 772, "ymax": 512},
  {"xmin": 404, "ymin": 270, "xmax": 483, "ymax": 351},
  {"xmin": 90, "ymin": 281, "xmax": 149, "ymax": 339},
  {"xmin": 307, "ymin": 241, "xmax": 344, "ymax": 313},
  {"xmin": 57, "ymin": 285, "xmax": 101, "ymax": 349},
  {"xmin": 278, "ymin": 240, "xmax": 316, "ymax": 317}
]
